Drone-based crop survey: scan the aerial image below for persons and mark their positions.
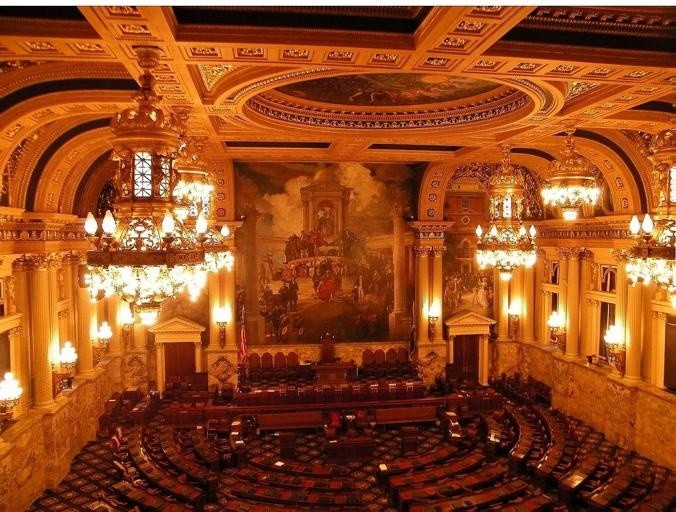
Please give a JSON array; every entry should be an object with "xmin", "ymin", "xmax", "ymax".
[
  {"xmin": 444, "ymin": 272, "xmax": 492, "ymax": 309},
  {"xmin": 262, "ymin": 227, "xmax": 395, "ymax": 337},
  {"xmin": 474, "ymin": 414, "xmax": 488, "ymax": 440}
]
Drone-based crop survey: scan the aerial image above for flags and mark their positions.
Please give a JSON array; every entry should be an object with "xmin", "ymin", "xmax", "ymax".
[
  {"xmin": 408, "ymin": 301, "xmax": 417, "ymax": 361},
  {"xmin": 240, "ymin": 306, "xmax": 248, "ymax": 364}
]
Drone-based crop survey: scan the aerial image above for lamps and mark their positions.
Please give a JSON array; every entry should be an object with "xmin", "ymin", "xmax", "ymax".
[
  {"xmin": 623, "ymin": 130, "xmax": 676, "ymax": 313},
  {"xmin": 53, "ymin": 340, "xmax": 78, "ymax": 396},
  {"xmin": 546, "ymin": 310, "xmax": 565, "ymax": 353},
  {"xmin": 475, "ymin": 119, "xmax": 601, "ymax": 284},
  {"xmin": 1, "ymin": 372, "xmax": 24, "ymax": 420},
  {"xmin": 603, "ymin": 323, "xmax": 625, "ymax": 373}
]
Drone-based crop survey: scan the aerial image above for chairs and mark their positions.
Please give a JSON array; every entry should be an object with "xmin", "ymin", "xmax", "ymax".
[
  {"xmin": 248, "ymin": 352, "xmax": 300, "ymax": 382},
  {"xmin": 362, "ymin": 348, "xmax": 412, "ymax": 376}
]
